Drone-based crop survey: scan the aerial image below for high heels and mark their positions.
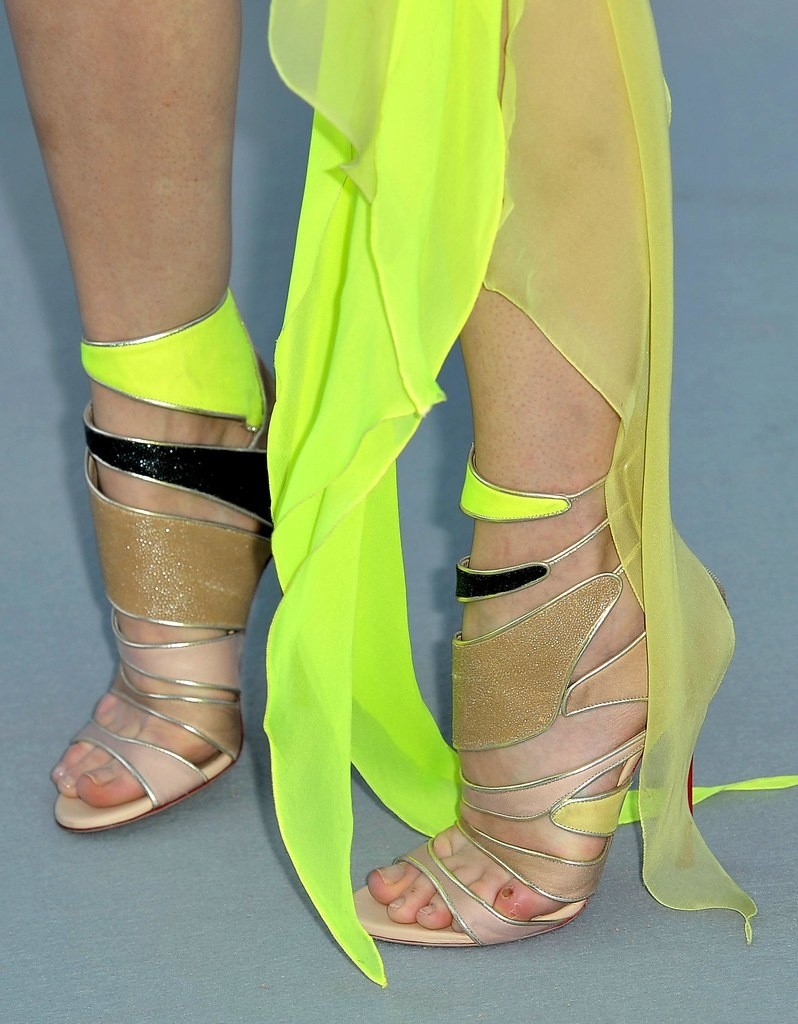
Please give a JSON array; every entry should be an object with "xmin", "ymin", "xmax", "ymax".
[
  {"xmin": 350, "ymin": 441, "xmax": 729, "ymax": 948},
  {"xmin": 49, "ymin": 288, "xmax": 271, "ymax": 833}
]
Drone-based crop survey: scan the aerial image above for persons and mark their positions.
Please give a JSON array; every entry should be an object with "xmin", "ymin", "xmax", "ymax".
[{"xmin": 0, "ymin": 0, "xmax": 798, "ymax": 987}]
